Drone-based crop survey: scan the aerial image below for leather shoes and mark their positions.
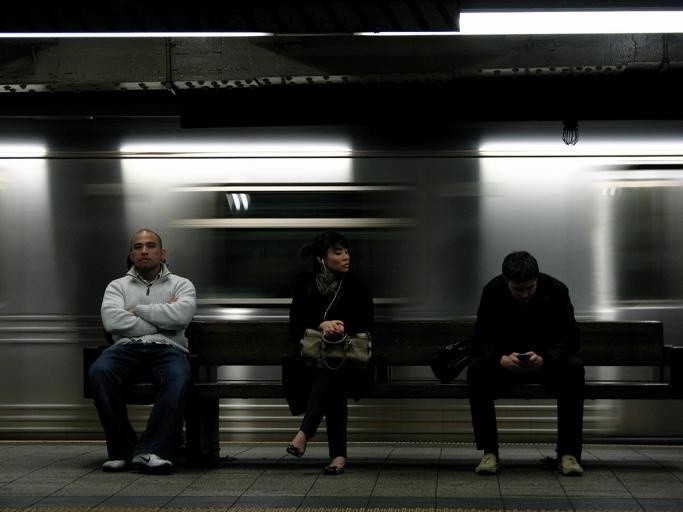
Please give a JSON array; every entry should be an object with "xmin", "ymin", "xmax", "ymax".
[
  {"xmin": 323, "ymin": 458, "xmax": 344, "ymax": 474},
  {"xmin": 286, "ymin": 440, "xmax": 307, "ymax": 457}
]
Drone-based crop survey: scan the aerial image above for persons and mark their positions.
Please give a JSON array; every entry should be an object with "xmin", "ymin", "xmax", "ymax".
[
  {"xmin": 281, "ymin": 229, "xmax": 375, "ymax": 474},
  {"xmin": 87, "ymin": 228, "xmax": 197, "ymax": 473},
  {"xmin": 467, "ymin": 251, "xmax": 585, "ymax": 476}
]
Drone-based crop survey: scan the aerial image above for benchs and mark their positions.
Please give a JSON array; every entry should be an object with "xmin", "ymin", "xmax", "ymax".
[{"xmin": 79, "ymin": 317, "xmax": 682, "ymax": 469}]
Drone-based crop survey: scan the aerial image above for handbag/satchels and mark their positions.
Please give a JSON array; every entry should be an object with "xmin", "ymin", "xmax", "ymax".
[{"xmin": 298, "ymin": 327, "xmax": 370, "ymax": 370}]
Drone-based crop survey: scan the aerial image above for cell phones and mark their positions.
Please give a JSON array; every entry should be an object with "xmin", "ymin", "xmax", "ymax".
[{"xmin": 516, "ymin": 354, "xmax": 530, "ymax": 364}]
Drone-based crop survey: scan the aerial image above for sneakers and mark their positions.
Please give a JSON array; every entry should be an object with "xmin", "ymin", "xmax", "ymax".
[
  {"xmin": 102, "ymin": 456, "xmax": 128, "ymax": 470},
  {"xmin": 130, "ymin": 452, "xmax": 173, "ymax": 474},
  {"xmin": 560, "ymin": 454, "xmax": 583, "ymax": 476},
  {"xmin": 474, "ymin": 453, "xmax": 498, "ymax": 474}
]
